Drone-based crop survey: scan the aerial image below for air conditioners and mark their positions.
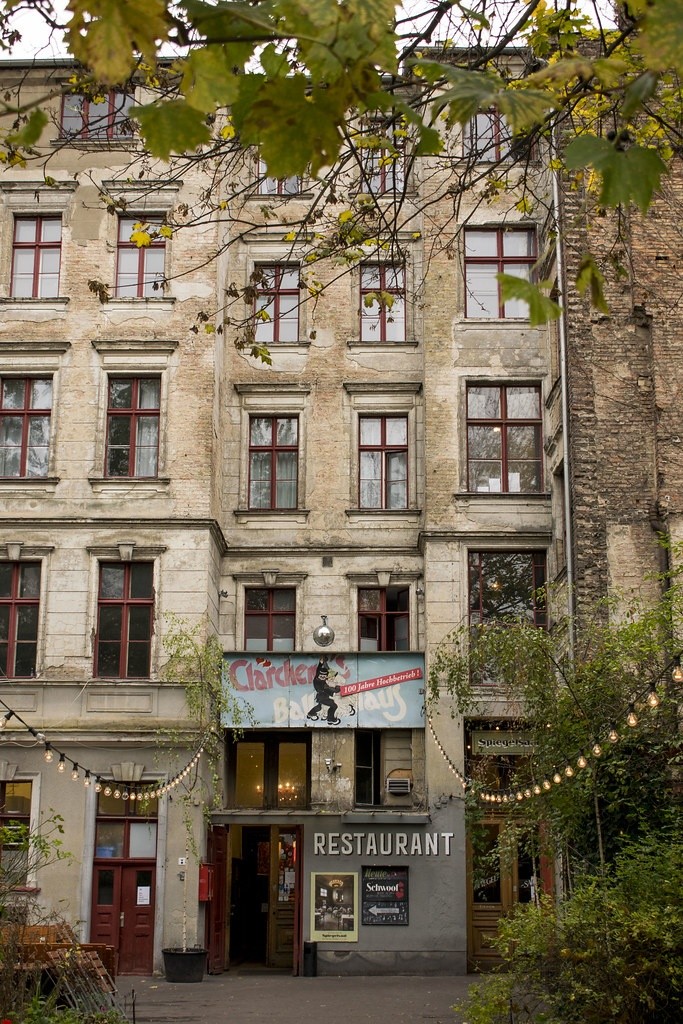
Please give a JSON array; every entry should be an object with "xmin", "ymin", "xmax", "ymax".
[{"xmin": 386, "ymin": 778, "xmax": 411, "ymax": 793}]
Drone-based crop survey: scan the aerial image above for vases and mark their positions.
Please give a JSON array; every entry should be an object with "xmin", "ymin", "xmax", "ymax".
[{"xmin": 161, "ymin": 947, "xmax": 209, "ymax": 983}]
